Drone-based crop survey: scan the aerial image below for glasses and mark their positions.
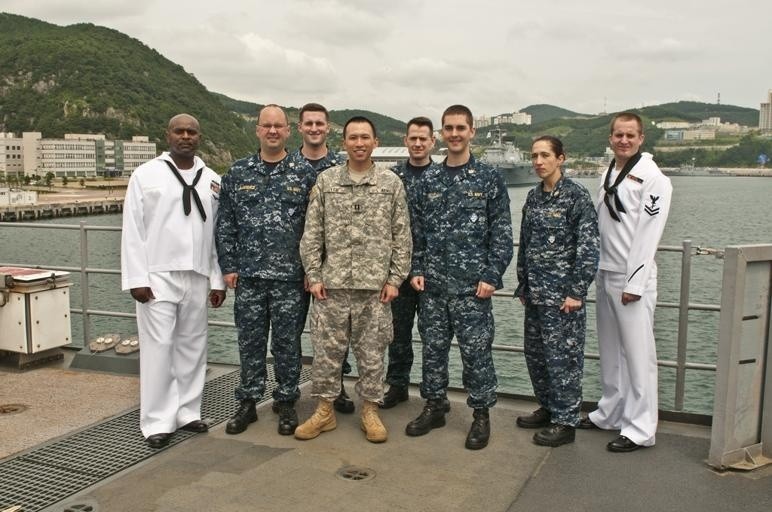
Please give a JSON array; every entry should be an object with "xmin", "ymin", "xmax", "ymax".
[{"xmin": 258, "ymin": 123, "xmax": 284, "ymax": 129}]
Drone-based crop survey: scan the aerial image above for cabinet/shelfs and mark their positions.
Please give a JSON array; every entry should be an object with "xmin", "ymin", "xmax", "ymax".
[{"xmin": 0, "ymin": 267, "xmax": 73, "ymax": 355}]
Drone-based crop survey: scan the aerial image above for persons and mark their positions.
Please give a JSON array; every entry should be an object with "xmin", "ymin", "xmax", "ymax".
[
  {"xmin": 574, "ymin": 113, "xmax": 673, "ymax": 453},
  {"xmin": 121, "ymin": 112, "xmax": 228, "ymax": 448},
  {"xmin": 294, "ymin": 115, "xmax": 414, "ymax": 444},
  {"xmin": 408, "ymin": 105, "xmax": 514, "ymax": 449},
  {"xmin": 379, "ymin": 117, "xmax": 451, "ymax": 414},
  {"xmin": 271, "ymin": 103, "xmax": 355, "ymax": 414},
  {"xmin": 216, "ymin": 105, "xmax": 319, "ymax": 436},
  {"xmin": 511, "ymin": 135, "xmax": 601, "ymax": 448}
]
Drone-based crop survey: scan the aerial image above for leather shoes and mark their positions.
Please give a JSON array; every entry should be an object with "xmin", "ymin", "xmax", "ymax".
[
  {"xmin": 146, "ymin": 434, "xmax": 170, "ymax": 448},
  {"xmin": 576, "ymin": 411, "xmax": 596, "ymax": 429},
  {"xmin": 180, "ymin": 421, "xmax": 208, "ymax": 432},
  {"xmin": 607, "ymin": 437, "xmax": 656, "ymax": 452}
]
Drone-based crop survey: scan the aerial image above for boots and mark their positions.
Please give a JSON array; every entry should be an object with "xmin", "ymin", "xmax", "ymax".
[
  {"xmin": 278, "ymin": 401, "xmax": 298, "ymax": 435},
  {"xmin": 406, "ymin": 399, "xmax": 445, "ymax": 436},
  {"xmin": 295, "ymin": 399, "xmax": 336, "ymax": 440},
  {"xmin": 334, "ymin": 390, "xmax": 353, "ymax": 413},
  {"xmin": 378, "ymin": 385, "xmax": 408, "ymax": 408},
  {"xmin": 360, "ymin": 400, "xmax": 388, "ymax": 442},
  {"xmin": 517, "ymin": 407, "xmax": 550, "ymax": 428},
  {"xmin": 227, "ymin": 399, "xmax": 256, "ymax": 434},
  {"xmin": 466, "ymin": 407, "xmax": 490, "ymax": 449},
  {"xmin": 534, "ymin": 422, "xmax": 575, "ymax": 447},
  {"xmin": 272, "ymin": 387, "xmax": 300, "ymax": 413}
]
